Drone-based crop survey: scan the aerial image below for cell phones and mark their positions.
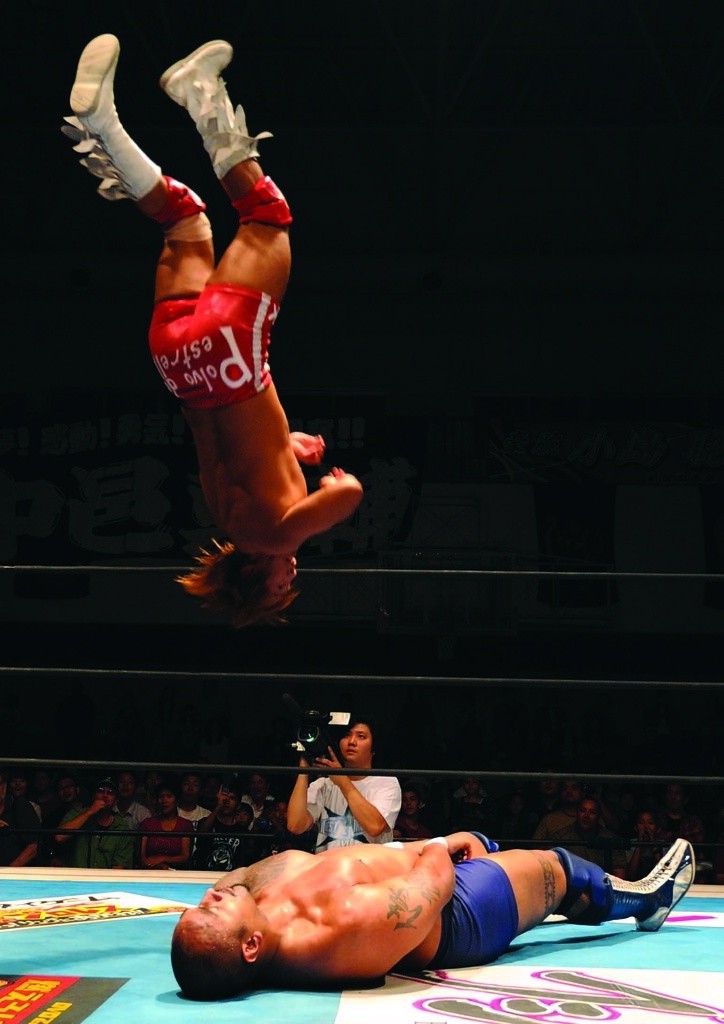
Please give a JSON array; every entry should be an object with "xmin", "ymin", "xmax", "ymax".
[{"xmin": 223, "ymin": 774, "xmax": 235, "ymax": 793}]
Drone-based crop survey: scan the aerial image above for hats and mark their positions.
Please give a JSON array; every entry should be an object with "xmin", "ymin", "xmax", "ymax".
[{"xmin": 95, "ymin": 777, "xmax": 118, "ymax": 789}]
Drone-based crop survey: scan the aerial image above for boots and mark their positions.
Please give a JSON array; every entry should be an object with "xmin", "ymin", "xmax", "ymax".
[
  {"xmin": 160, "ymin": 39, "xmax": 272, "ymax": 179},
  {"xmin": 61, "ymin": 34, "xmax": 161, "ymax": 199},
  {"xmin": 550, "ymin": 838, "xmax": 696, "ymax": 932}
]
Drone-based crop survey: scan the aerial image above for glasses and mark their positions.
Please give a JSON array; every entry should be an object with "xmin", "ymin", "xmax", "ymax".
[{"xmin": 97, "ymin": 788, "xmax": 114, "ymax": 795}]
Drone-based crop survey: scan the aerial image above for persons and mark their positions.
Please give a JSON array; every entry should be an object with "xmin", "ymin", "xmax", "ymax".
[
  {"xmin": 171, "ymin": 832, "xmax": 696, "ymax": 998},
  {"xmin": 0, "ymin": 769, "xmax": 702, "ymax": 869},
  {"xmin": 287, "ymin": 719, "xmax": 401, "ymax": 853},
  {"xmin": 69, "ymin": 32, "xmax": 364, "ymax": 617}
]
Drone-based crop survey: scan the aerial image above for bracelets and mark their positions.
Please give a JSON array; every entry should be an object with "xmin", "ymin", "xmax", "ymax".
[{"xmin": 424, "ymin": 837, "xmax": 448, "ymax": 847}]
